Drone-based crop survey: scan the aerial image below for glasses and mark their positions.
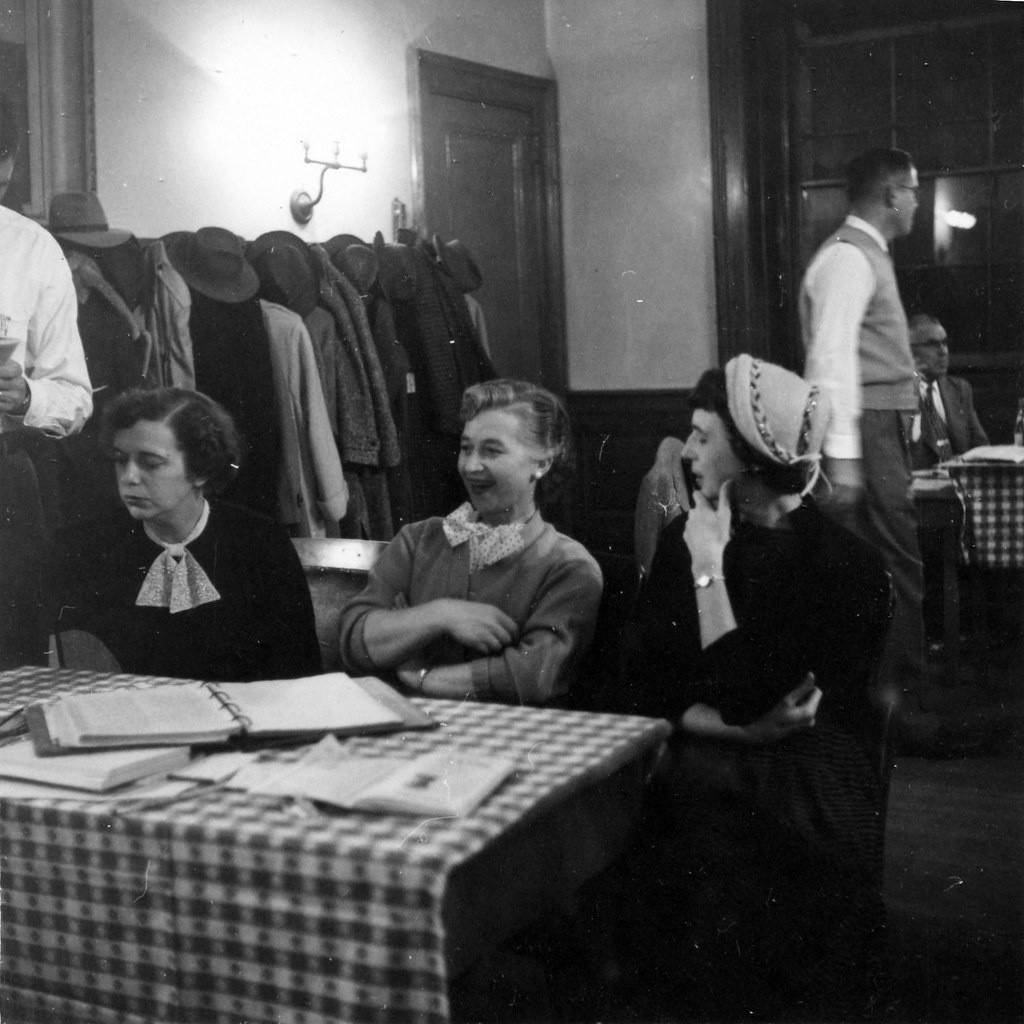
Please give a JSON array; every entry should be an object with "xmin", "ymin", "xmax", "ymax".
[
  {"xmin": 911, "ymin": 339, "xmax": 949, "ymax": 349},
  {"xmin": 897, "ymin": 184, "xmax": 922, "ymax": 200}
]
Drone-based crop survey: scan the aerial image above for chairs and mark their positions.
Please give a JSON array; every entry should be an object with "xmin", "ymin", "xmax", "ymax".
[{"xmin": 291, "ymin": 537, "xmax": 389, "ymax": 674}]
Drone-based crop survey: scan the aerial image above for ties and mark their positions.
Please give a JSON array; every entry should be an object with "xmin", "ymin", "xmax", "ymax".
[{"xmin": 924, "ymin": 384, "xmax": 951, "ymax": 462}]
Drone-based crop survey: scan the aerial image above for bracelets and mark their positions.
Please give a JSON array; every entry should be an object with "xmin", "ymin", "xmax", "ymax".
[{"xmin": 418, "ymin": 664, "xmax": 432, "ymax": 691}]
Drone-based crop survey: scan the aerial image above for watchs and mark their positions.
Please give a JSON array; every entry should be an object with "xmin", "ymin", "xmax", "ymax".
[{"xmin": 695, "ymin": 576, "xmax": 725, "ymax": 588}]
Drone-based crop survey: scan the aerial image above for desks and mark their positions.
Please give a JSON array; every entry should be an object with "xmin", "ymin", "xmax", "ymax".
[
  {"xmin": 0, "ymin": 664, "xmax": 673, "ymax": 1024},
  {"xmin": 932, "ymin": 443, "xmax": 1024, "ymax": 670}
]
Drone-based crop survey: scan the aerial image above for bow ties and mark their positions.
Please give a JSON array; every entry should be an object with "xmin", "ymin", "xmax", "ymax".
[{"xmin": 444, "ymin": 501, "xmax": 525, "ymax": 565}]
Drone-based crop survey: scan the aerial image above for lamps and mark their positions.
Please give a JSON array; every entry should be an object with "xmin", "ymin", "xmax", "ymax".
[{"xmin": 290, "ymin": 137, "xmax": 367, "ymax": 225}]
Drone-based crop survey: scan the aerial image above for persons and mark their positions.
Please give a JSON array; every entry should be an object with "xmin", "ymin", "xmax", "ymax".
[
  {"xmin": 341, "ymin": 378, "xmax": 602, "ymax": 704},
  {"xmin": 907, "ymin": 313, "xmax": 987, "ymax": 470},
  {"xmin": 588, "ymin": 353, "xmax": 896, "ymax": 869},
  {"xmin": 793, "ymin": 146, "xmax": 987, "ymax": 758},
  {"xmin": 0, "ymin": 91, "xmax": 92, "ymax": 668},
  {"xmin": 34, "ymin": 389, "xmax": 321, "ymax": 676}
]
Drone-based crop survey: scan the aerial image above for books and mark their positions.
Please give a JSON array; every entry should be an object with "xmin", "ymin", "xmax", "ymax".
[
  {"xmin": 27, "ymin": 671, "xmax": 438, "ymax": 756},
  {"xmin": 0, "ymin": 740, "xmax": 190, "ymax": 791},
  {"xmin": 251, "ymin": 735, "xmax": 518, "ymax": 818}
]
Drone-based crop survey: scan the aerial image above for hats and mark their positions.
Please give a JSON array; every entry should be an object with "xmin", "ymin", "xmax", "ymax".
[
  {"xmin": 247, "ymin": 230, "xmax": 319, "ymax": 315},
  {"xmin": 374, "ymin": 231, "xmax": 415, "ymax": 303},
  {"xmin": 45, "ymin": 191, "xmax": 132, "ymax": 250},
  {"xmin": 725, "ymin": 354, "xmax": 829, "ymax": 497},
  {"xmin": 433, "ymin": 234, "xmax": 483, "ymax": 298},
  {"xmin": 158, "ymin": 227, "xmax": 260, "ymax": 304},
  {"xmin": 323, "ymin": 234, "xmax": 379, "ymax": 298}
]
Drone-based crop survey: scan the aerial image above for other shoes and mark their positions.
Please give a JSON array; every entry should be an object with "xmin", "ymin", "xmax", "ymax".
[{"xmin": 895, "ymin": 728, "xmax": 992, "ymax": 758}]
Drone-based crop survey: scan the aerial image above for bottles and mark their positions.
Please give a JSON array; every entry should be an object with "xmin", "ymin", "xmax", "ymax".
[{"xmin": 1014, "ymin": 398, "xmax": 1024, "ymax": 446}]
